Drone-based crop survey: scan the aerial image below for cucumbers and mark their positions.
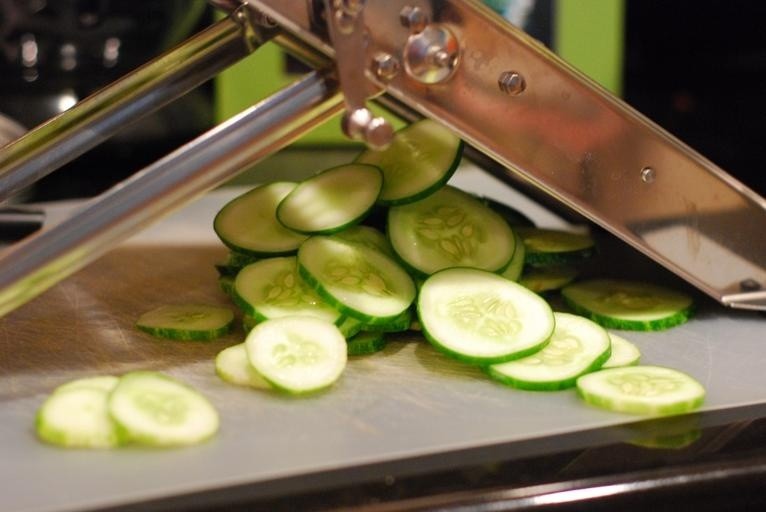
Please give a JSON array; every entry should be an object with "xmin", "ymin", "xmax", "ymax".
[{"xmin": 36, "ymin": 116, "xmax": 709, "ymax": 445}]
